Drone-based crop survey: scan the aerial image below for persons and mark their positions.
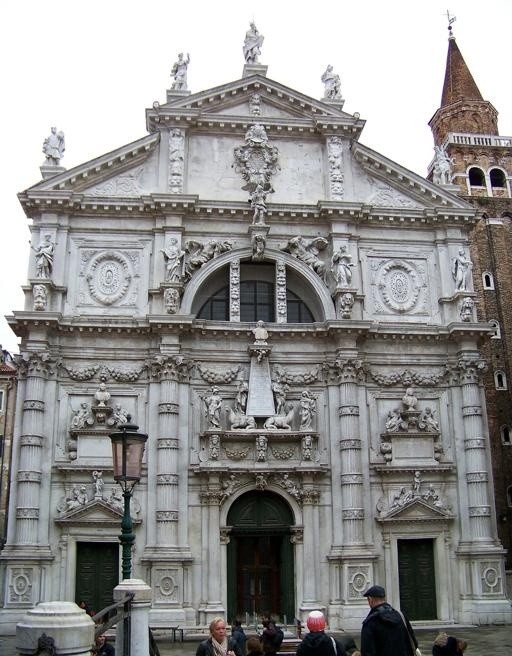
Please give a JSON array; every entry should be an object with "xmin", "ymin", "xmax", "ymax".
[
  {"xmin": 243, "ymin": 21, "xmax": 264, "ymax": 64},
  {"xmin": 322, "ymin": 65, "xmax": 342, "ymax": 100},
  {"xmin": 332, "ymin": 243, "xmax": 355, "ymax": 286},
  {"xmin": 91, "ymin": 632, "xmax": 114, "ymax": 656},
  {"xmin": 455, "ymin": 248, "xmax": 470, "ymax": 291},
  {"xmin": 170, "ymin": 53, "xmax": 190, "ymax": 91},
  {"xmin": 41, "ymin": 126, "xmax": 66, "ymax": 165},
  {"xmin": 300, "ymin": 390, "xmax": 316, "ymax": 429},
  {"xmin": 159, "ymin": 237, "xmax": 186, "ymax": 283},
  {"xmin": 434, "ymin": 146, "xmax": 452, "ymax": 184},
  {"xmin": 361, "ymin": 585, "xmax": 423, "ymax": 655},
  {"xmin": 29, "ymin": 232, "xmax": 55, "ymax": 279},
  {"xmin": 202, "ymin": 388, "xmax": 222, "ymax": 428},
  {"xmin": 196, "ymin": 611, "xmax": 284, "ymax": 656},
  {"xmin": 295, "ymin": 610, "xmax": 350, "ymax": 655},
  {"xmin": 252, "ymin": 185, "xmax": 270, "ymax": 226}
]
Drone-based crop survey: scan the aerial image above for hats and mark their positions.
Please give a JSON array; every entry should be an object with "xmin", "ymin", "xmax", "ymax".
[
  {"xmin": 363, "ymin": 585, "xmax": 385, "ymax": 597},
  {"xmin": 307, "ymin": 611, "xmax": 325, "ymax": 631},
  {"xmin": 432, "ymin": 632, "xmax": 467, "ymax": 656}
]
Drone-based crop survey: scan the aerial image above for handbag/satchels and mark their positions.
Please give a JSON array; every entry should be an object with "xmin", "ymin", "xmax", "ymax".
[{"xmin": 414, "ymin": 648, "xmax": 422, "ymax": 656}]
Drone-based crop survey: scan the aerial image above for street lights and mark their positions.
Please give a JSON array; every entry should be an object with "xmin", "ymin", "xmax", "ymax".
[{"xmin": 108, "ymin": 413, "xmax": 148, "ymax": 581}]
